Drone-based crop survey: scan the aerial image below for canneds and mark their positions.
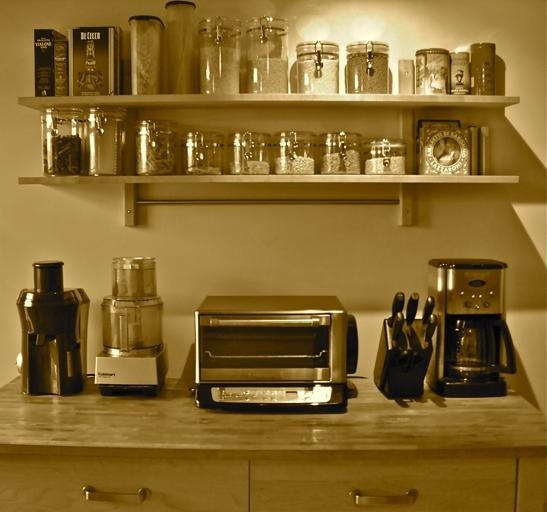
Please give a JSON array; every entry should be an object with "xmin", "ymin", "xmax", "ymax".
[{"xmin": 416, "ymin": 47, "xmax": 451, "ymax": 95}]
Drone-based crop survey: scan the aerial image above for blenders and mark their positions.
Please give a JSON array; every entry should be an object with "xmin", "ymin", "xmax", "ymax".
[{"xmin": 94, "ymin": 256, "xmax": 167, "ymax": 397}]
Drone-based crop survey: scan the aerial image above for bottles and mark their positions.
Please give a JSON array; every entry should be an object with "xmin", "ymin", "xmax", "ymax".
[
  {"xmin": 182, "ymin": 129, "xmax": 223, "ymax": 175},
  {"xmin": 274, "ymin": 132, "xmax": 314, "ymax": 173},
  {"xmin": 65, "ymin": 159, "xmax": 69, "ymax": 161},
  {"xmin": 198, "ymin": 17, "xmax": 239, "ymax": 96},
  {"xmin": 41, "ymin": 107, "xmax": 82, "ymax": 174},
  {"xmin": 411, "ymin": 49, "xmax": 450, "ymax": 93},
  {"xmin": 470, "ymin": 43, "xmax": 495, "ymax": 96},
  {"xmin": 241, "ymin": 17, "xmax": 288, "ymax": 93},
  {"xmin": 128, "ymin": 15, "xmax": 163, "ymax": 95},
  {"xmin": 397, "ymin": 59, "xmax": 415, "ymax": 93},
  {"xmin": 450, "ymin": 51, "xmax": 470, "ymax": 93},
  {"xmin": 136, "ymin": 120, "xmax": 175, "ymax": 174},
  {"xmin": 228, "ymin": 129, "xmax": 271, "ymax": 175},
  {"xmin": 295, "ymin": 42, "xmax": 339, "ymax": 93},
  {"xmin": 164, "ymin": 1, "xmax": 195, "ymax": 94},
  {"xmin": 346, "ymin": 41, "xmax": 388, "ymax": 93},
  {"xmin": 364, "ymin": 139, "xmax": 405, "ymax": 174},
  {"xmin": 467, "ymin": 126, "xmax": 490, "ymax": 176},
  {"xmin": 318, "ymin": 132, "xmax": 362, "ymax": 173},
  {"xmin": 86, "ymin": 107, "xmax": 125, "ymax": 177}
]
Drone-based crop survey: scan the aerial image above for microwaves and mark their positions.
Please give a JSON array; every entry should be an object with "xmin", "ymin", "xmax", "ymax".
[{"xmin": 195, "ymin": 295, "xmax": 358, "ymax": 411}]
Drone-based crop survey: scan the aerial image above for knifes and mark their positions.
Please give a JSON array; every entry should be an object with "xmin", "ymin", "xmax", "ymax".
[
  {"xmin": 387, "ymin": 291, "xmax": 405, "ymax": 328},
  {"xmin": 407, "ymin": 292, "xmax": 418, "ymax": 326},
  {"xmin": 424, "ymin": 314, "xmax": 438, "ymax": 342},
  {"xmin": 390, "ymin": 312, "xmax": 403, "ymax": 340},
  {"xmin": 422, "ymin": 295, "xmax": 435, "ymax": 325}
]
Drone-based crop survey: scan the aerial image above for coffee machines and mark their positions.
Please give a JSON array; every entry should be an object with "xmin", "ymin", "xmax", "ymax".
[
  {"xmin": 430, "ymin": 258, "xmax": 515, "ymax": 394},
  {"xmin": 16, "ymin": 262, "xmax": 90, "ymax": 396}
]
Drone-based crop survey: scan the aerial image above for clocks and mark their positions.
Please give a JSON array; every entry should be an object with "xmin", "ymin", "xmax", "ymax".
[{"xmin": 419, "ymin": 126, "xmax": 471, "ymax": 176}]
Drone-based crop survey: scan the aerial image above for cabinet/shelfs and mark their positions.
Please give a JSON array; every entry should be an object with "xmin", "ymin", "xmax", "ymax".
[
  {"xmin": 0, "ymin": 449, "xmax": 250, "ymax": 512},
  {"xmin": 16, "ymin": 95, "xmax": 521, "ymax": 226},
  {"xmin": 249, "ymin": 449, "xmax": 547, "ymax": 512}
]
